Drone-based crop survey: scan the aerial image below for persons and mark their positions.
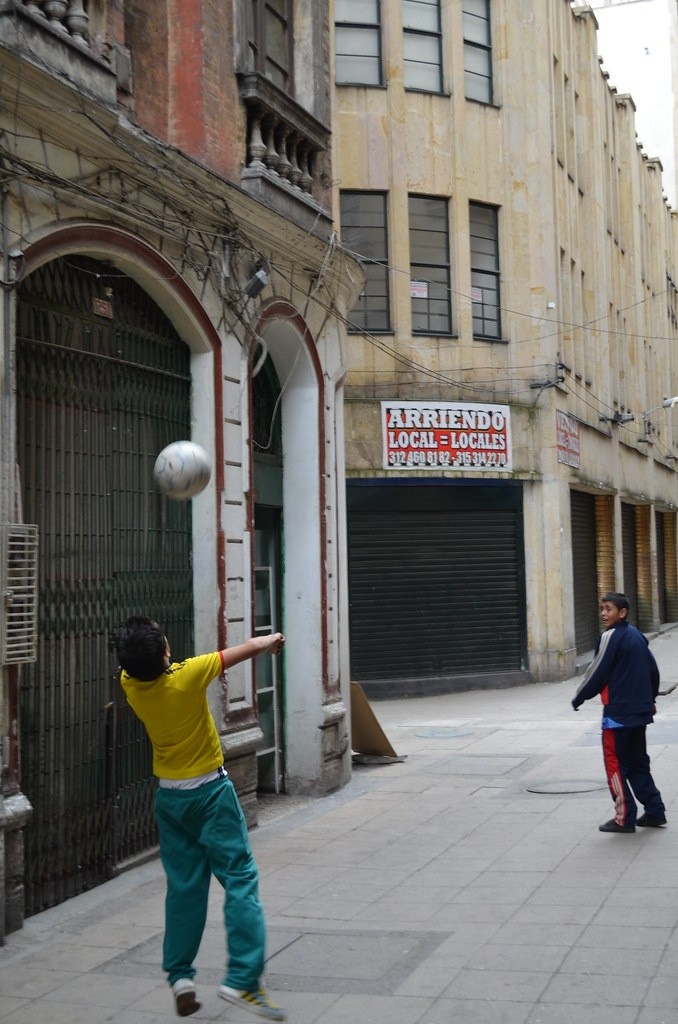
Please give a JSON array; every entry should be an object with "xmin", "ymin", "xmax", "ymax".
[
  {"xmin": 570, "ymin": 591, "xmax": 669, "ymax": 833},
  {"xmin": 113, "ymin": 614, "xmax": 293, "ymax": 1022}
]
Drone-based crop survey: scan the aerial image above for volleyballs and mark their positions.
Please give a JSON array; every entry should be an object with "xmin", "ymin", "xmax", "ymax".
[{"xmin": 154, "ymin": 440, "xmax": 212, "ymax": 502}]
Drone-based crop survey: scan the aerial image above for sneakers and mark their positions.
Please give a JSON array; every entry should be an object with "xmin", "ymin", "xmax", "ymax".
[
  {"xmin": 172, "ymin": 978, "xmax": 203, "ymax": 1019},
  {"xmin": 601, "ymin": 815, "xmax": 634, "ymax": 833},
  {"xmin": 638, "ymin": 809, "xmax": 669, "ymax": 829},
  {"xmin": 220, "ymin": 977, "xmax": 288, "ymax": 1019}
]
